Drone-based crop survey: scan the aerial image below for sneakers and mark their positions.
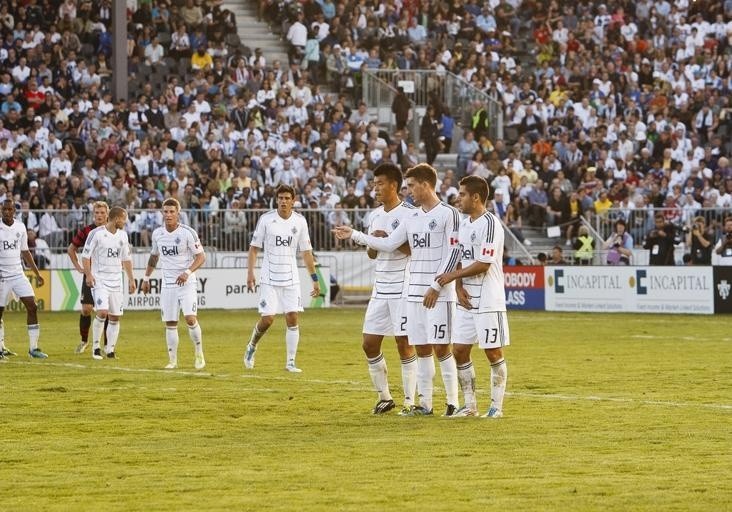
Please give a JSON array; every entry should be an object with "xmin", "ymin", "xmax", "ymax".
[
  {"xmin": 106, "ymin": 352, "xmax": 120, "ymax": 360},
  {"xmin": 164, "ymin": 360, "xmax": 178, "ymax": 369},
  {"xmin": 243, "ymin": 343, "xmax": 257, "ymax": 369},
  {"xmin": 27, "ymin": 348, "xmax": 49, "ymax": 359},
  {"xmin": 285, "ymin": 365, "xmax": 302, "ymax": 373},
  {"xmin": 372, "ymin": 400, "xmax": 395, "ymax": 414},
  {"xmin": 93, "ymin": 346, "xmax": 104, "ymax": 360},
  {"xmin": 75, "ymin": 340, "xmax": 89, "ymax": 354},
  {"xmin": 195, "ymin": 352, "xmax": 205, "ymax": 369},
  {"xmin": 0, "ymin": 347, "xmax": 18, "ymax": 360},
  {"xmin": 397, "ymin": 403, "xmax": 503, "ymax": 418}
]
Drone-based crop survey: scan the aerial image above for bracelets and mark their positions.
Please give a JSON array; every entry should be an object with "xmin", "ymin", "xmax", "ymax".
[
  {"xmin": 184, "ymin": 268, "xmax": 191, "ymax": 276},
  {"xmin": 144, "ymin": 275, "xmax": 151, "ymax": 281},
  {"xmin": 311, "ymin": 273, "xmax": 318, "ymax": 282},
  {"xmin": 430, "ymin": 280, "xmax": 441, "ymax": 291}
]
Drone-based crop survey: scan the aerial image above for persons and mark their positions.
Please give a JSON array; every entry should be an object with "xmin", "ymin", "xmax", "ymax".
[
  {"xmin": 360, "ymin": 163, "xmax": 410, "ymax": 415},
  {"xmin": 0, "ymin": 1, "xmax": 731, "ymax": 267},
  {"xmin": 243, "ymin": 185, "xmax": 320, "ymax": 372},
  {"xmin": 84, "ymin": 206, "xmax": 136, "ymax": 359},
  {"xmin": 434, "ymin": 175, "xmax": 510, "ymax": 418},
  {"xmin": 330, "ymin": 163, "xmax": 458, "ymax": 416},
  {"xmin": 141, "ymin": 198, "xmax": 205, "ymax": 369},
  {"xmin": 68, "ymin": 201, "xmax": 111, "ymax": 353},
  {"xmin": 0, "ymin": 198, "xmax": 49, "ymax": 360}
]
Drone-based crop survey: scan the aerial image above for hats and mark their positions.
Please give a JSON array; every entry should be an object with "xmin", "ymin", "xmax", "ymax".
[
  {"xmin": 634, "ymin": 131, "xmax": 647, "ymax": 141},
  {"xmin": 29, "ymin": 181, "xmax": 39, "ymax": 188},
  {"xmin": 324, "ymin": 182, "xmax": 332, "ymax": 190},
  {"xmin": 34, "ymin": 116, "xmax": 42, "ymax": 123},
  {"xmin": 593, "ymin": 78, "xmax": 601, "ymax": 85},
  {"xmin": 703, "ymin": 167, "xmax": 713, "ymax": 179},
  {"xmin": 313, "ymin": 147, "xmax": 322, "ymax": 154},
  {"xmin": 535, "ymin": 98, "xmax": 543, "ymax": 103}
]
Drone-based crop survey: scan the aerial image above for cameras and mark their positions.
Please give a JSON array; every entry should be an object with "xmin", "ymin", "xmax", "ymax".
[
  {"xmin": 649, "ymin": 230, "xmax": 660, "ymax": 239},
  {"xmin": 722, "ymin": 230, "xmax": 732, "ymax": 239},
  {"xmin": 615, "ymin": 235, "xmax": 622, "ymax": 247},
  {"xmin": 693, "ymin": 224, "xmax": 702, "ymax": 229}
]
